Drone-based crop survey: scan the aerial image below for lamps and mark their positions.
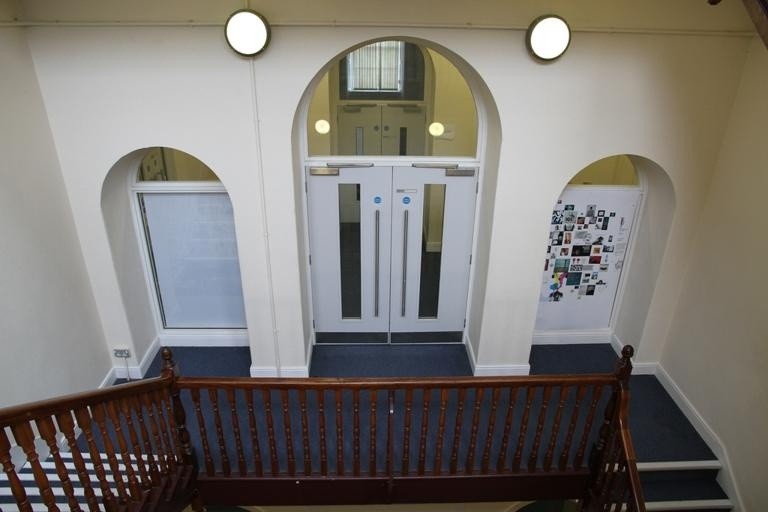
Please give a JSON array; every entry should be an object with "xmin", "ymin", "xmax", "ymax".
[
  {"xmin": 526, "ymin": 12, "xmax": 572, "ymax": 62},
  {"xmin": 222, "ymin": 10, "xmax": 269, "ymax": 61}
]
